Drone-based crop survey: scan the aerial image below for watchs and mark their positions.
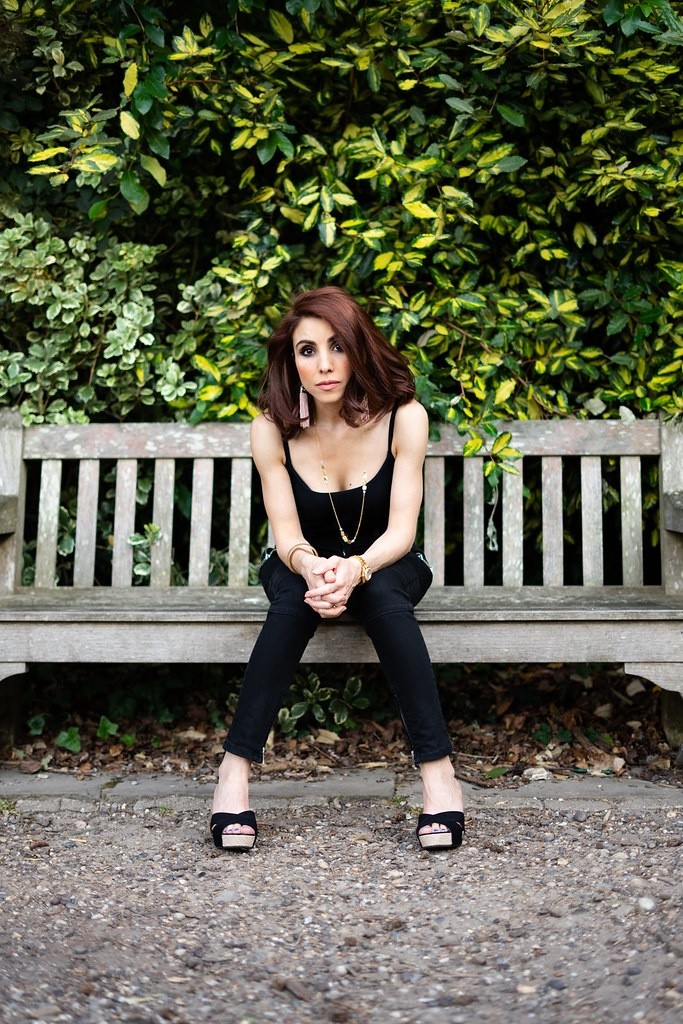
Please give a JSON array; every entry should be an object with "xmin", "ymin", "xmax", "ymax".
[{"xmin": 351, "ymin": 555, "xmax": 372, "ymax": 585}]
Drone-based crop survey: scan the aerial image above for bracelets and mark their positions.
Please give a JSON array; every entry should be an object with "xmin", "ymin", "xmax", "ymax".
[{"xmin": 287, "ymin": 543, "xmax": 318, "ymax": 574}]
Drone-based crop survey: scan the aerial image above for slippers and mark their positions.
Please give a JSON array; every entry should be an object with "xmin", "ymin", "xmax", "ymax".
[
  {"xmin": 209, "ymin": 809, "xmax": 258, "ymax": 849},
  {"xmin": 416, "ymin": 811, "xmax": 464, "ymax": 853}
]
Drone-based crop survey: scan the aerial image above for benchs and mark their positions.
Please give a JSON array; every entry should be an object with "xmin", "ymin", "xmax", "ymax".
[{"xmin": 0, "ymin": 407, "xmax": 683, "ymax": 697}]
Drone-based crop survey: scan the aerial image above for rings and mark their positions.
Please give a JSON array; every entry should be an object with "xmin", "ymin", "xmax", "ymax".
[{"xmin": 333, "ymin": 605, "xmax": 335, "ymax": 609}]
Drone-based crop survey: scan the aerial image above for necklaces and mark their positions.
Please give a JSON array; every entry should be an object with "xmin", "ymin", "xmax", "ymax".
[{"xmin": 312, "ymin": 417, "xmax": 367, "ymax": 545}]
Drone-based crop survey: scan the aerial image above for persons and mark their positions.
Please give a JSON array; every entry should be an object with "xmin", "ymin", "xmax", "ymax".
[{"xmin": 207, "ymin": 285, "xmax": 466, "ymax": 848}]
